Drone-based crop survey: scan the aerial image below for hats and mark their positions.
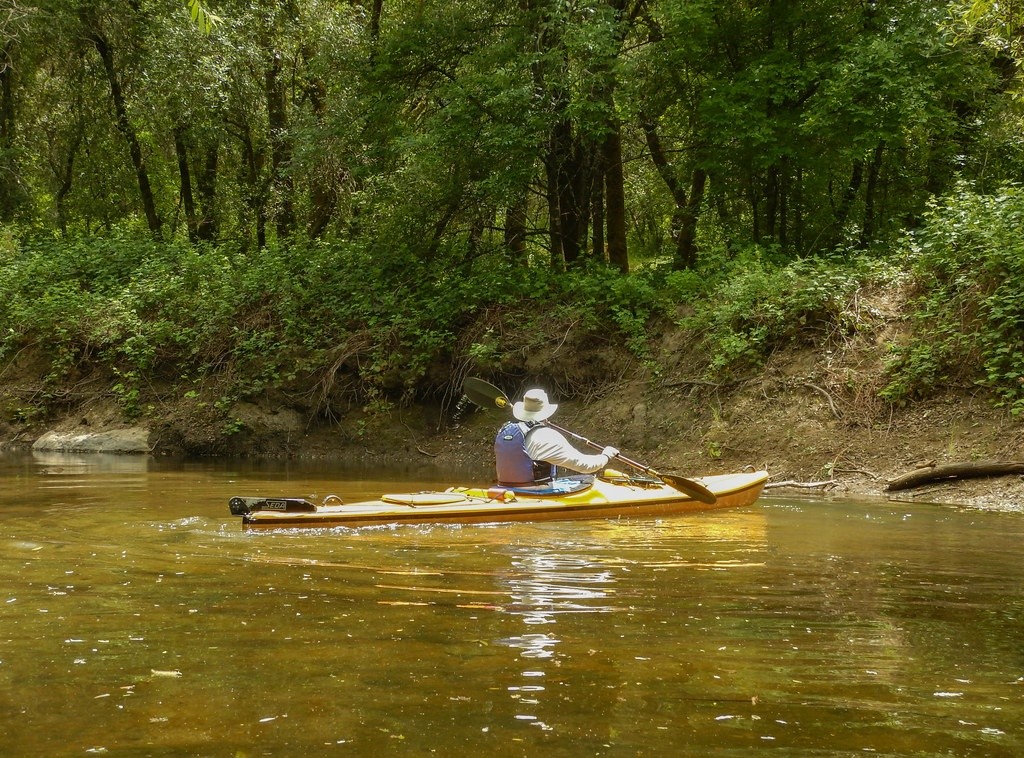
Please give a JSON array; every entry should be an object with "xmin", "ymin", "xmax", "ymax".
[{"xmin": 513, "ymin": 389, "xmax": 558, "ymax": 421}]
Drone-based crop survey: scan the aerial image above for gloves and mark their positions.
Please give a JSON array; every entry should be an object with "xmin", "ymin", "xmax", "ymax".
[{"xmin": 602, "ymin": 446, "xmax": 619, "ymax": 462}]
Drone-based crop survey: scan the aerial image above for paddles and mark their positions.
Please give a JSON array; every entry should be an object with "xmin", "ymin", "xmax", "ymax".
[{"xmin": 462, "ymin": 375, "xmax": 718, "ymax": 506}]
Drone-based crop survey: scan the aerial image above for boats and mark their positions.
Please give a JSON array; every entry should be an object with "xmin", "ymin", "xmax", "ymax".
[{"xmin": 227, "ymin": 471, "xmax": 769, "ymax": 533}]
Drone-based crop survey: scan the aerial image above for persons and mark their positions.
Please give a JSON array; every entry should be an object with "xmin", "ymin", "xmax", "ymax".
[{"xmin": 493, "ymin": 388, "xmax": 620, "ymax": 486}]
